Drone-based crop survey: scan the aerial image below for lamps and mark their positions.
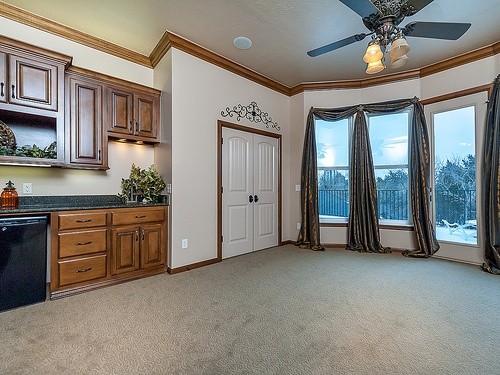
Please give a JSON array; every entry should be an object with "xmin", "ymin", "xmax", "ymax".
[{"xmin": 362, "ymin": 0, "xmax": 409, "ymax": 74}]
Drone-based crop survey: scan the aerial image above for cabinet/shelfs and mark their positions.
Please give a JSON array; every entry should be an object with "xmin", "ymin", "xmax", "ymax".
[
  {"xmin": 49, "ymin": 209, "xmax": 109, "ymax": 300},
  {"xmin": 64, "ymin": 65, "xmax": 110, "ymax": 171},
  {"xmin": 109, "ymin": 205, "xmax": 168, "ymax": 286},
  {"xmin": 108, "ymin": 75, "xmax": 161, "ymax": 144},
  {"xmin": 0, "ymin": 34, "xmax": 73, "ymax": 167}
]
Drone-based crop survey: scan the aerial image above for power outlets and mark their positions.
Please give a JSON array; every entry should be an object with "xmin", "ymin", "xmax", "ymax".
[
  {"xmin": 181, "ymin": 239, "xmax": 188, "ymax": 249},
  {"xmin": 23, "ymin": 184, "xmax": 32, "ymax": 194},
  {"xmin": 295, "ymin": 185, "xmax": 300, "ymax": 191}
]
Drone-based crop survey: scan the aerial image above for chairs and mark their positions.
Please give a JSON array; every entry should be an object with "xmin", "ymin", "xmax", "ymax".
[{"xmin": 442, "ymin": 219, "xmax": 477, "ymax": 241}]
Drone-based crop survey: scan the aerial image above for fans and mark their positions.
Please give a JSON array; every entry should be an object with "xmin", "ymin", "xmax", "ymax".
[{"xmin": 308, "ymin": 0, "xmax": 471, "ymax": 57}]
